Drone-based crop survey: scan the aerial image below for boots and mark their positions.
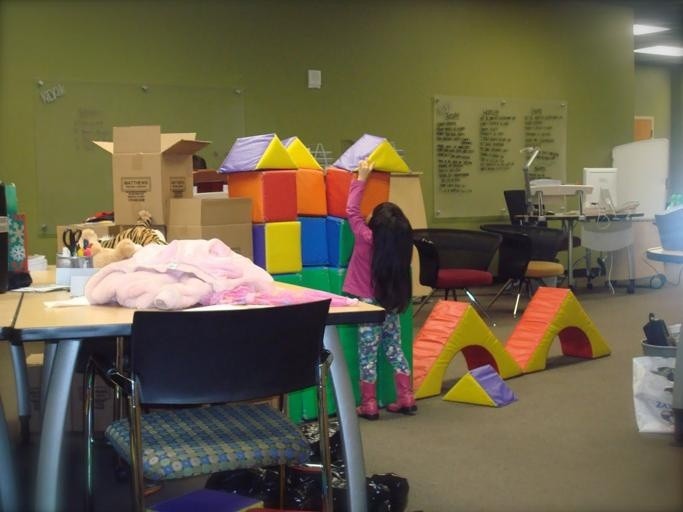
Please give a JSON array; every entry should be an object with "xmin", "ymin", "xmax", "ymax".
[
  {"xmin": 356, "ymin": 380, "xmax": 380, "ymax": 420},
  {"xmin": 387, "ymin": 373, "xmax": 417, "ymax": 412}
]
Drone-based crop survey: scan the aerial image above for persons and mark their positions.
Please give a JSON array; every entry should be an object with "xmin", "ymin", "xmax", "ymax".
[{"xmin": 343, "ymin": 159, "xmax": 418, "ymax": 420}]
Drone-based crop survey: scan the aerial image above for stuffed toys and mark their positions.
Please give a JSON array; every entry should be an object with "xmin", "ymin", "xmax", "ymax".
[
  {"xmin": 97, "ymin": 209, "xmax": 162, "ymax": 248},
  {"xmin": 76, "ymin": 230, "xmax": 136, "ymax": 268}
]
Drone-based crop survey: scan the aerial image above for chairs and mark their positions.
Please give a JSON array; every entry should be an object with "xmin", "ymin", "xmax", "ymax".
[{"xmin": 409, "ymin": 189, "xmax": 581, "ymax": 328}]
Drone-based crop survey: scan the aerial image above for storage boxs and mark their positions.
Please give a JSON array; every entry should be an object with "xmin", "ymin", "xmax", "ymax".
[{"xmin": 56, "ymin": 124, "xmax": 254, "ymax": 268}]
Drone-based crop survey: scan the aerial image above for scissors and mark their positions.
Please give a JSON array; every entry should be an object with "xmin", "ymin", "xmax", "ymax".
[{"xmin": 63, "ymin": 229, "xmax": 82, "ymax": 255}]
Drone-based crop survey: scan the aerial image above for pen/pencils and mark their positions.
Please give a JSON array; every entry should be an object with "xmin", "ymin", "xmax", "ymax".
[{"xmin": 63, "ymin": 239, "xmax": 91, "ymax": 256}]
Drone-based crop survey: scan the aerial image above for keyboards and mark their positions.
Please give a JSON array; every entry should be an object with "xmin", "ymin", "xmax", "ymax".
[{"xmin": 563, "ymin": 211, "xmax": 579, "ymax": 214}]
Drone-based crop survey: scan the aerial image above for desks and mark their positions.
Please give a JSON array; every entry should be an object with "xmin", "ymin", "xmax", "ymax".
[{"xmin": 514, "ymin": 209, "xmax": 644, "ymax": 296}]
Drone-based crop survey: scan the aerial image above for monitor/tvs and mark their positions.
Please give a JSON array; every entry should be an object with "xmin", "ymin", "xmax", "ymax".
[{"xmin": 583, "ymin": 168, "xmax": 619, "ymax": 208}]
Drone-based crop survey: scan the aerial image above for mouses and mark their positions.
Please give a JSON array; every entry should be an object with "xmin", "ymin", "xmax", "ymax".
[{"xmin": 544, "ymin": 211, "xmax": 555, "ymax": 215}]
